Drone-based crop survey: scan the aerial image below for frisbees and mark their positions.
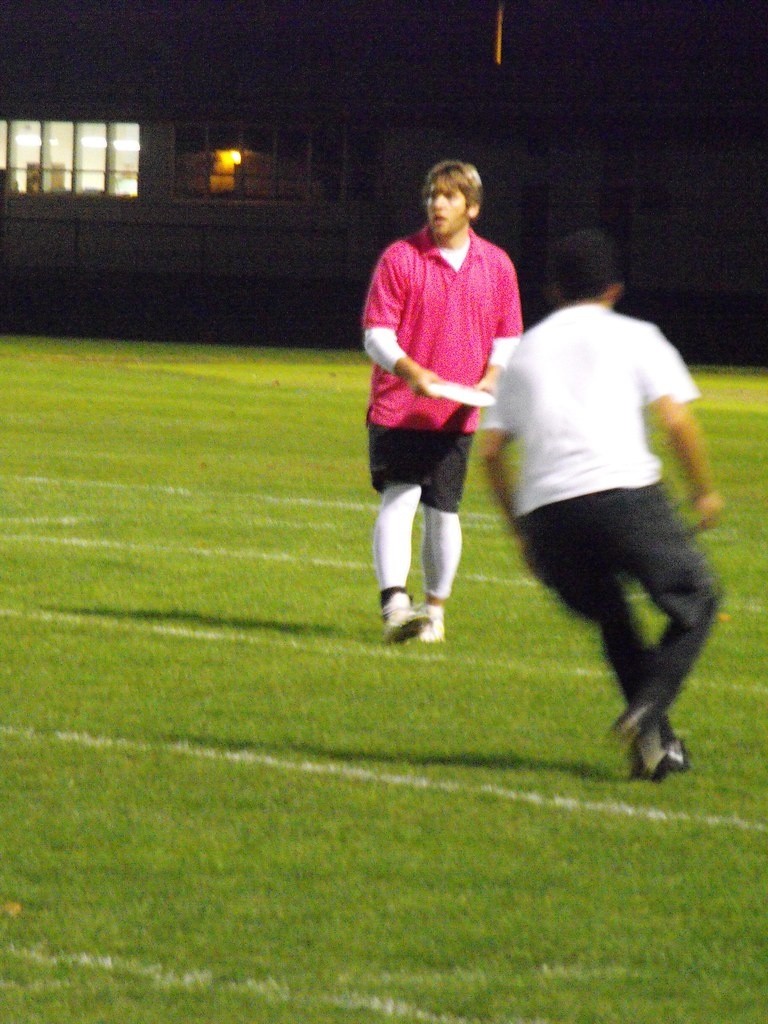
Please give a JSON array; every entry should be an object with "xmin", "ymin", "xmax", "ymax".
[{"xmin": 422, "ymin": 379, "xmax": 494, "ymax": 410}]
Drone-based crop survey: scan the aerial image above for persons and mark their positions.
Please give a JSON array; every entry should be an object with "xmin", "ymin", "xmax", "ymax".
[
  {"xmin": 482, "ymin": 220, "xmax": 727, "ymax": 783},
  {"xmin": 362, "ymin": 160, "xmax": 524, "ymax": 642}
]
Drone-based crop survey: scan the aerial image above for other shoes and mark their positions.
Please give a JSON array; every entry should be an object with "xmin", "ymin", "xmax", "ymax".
[
  {"xmin": 416, "ymin": 604, "xmax": 445, "ymax": 643},
  {"xmin": 615, "ymin": 696, "xmax": 685, "ymax": 783},
  {"xmin": 379, "ymin": 608, "xmax": 430, "ymax": 646}
]
